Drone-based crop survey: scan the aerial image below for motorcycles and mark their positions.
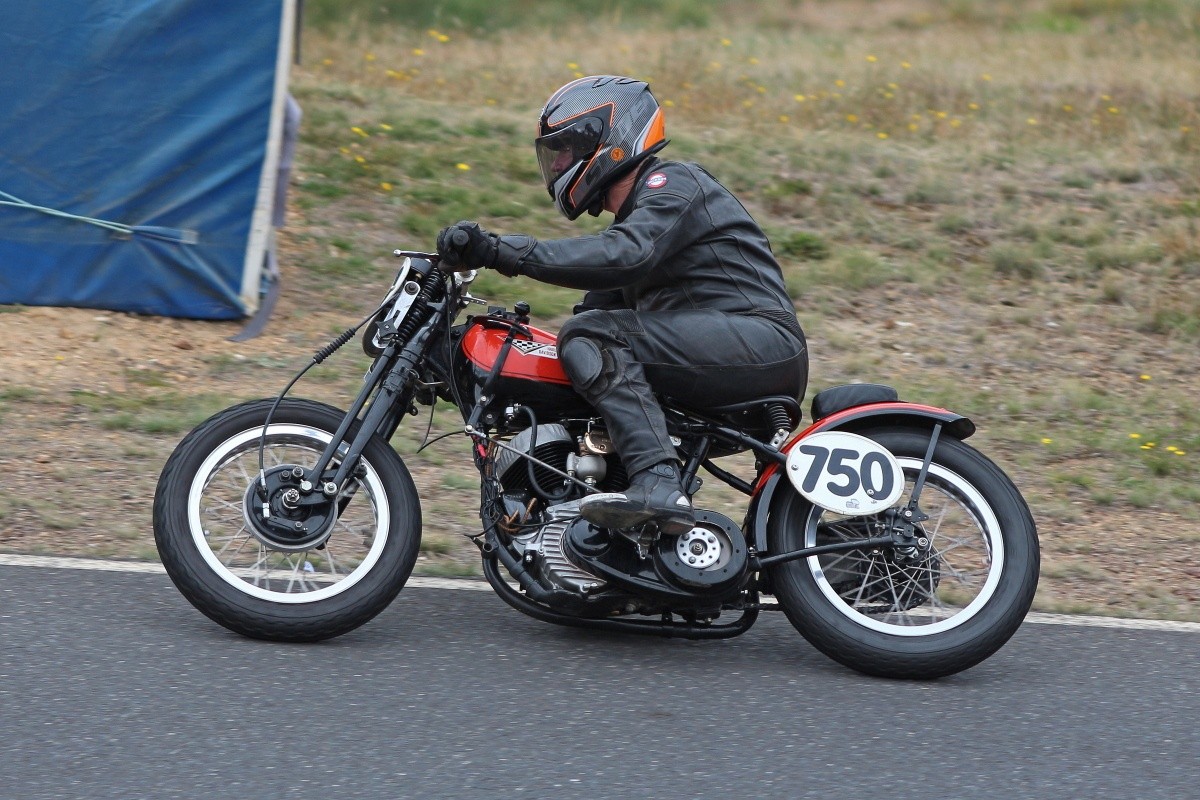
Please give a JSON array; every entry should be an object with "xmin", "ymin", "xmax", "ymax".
[{"xmin": 151, "ymin": 228, "xmax": 1042, "ymax": 682}]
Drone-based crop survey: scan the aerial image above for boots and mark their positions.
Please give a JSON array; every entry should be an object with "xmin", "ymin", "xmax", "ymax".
[{"xmin": 579, "ymin": 457, "xmax": 696, "ymax": 534}]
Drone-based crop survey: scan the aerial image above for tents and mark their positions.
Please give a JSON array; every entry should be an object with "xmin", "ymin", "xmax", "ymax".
[{"xmin": 0, "ymin": 0, "xmax": 302, "ymax": 321}]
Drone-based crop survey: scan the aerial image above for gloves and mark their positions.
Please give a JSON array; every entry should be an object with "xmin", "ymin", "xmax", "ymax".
[{"xmin": 435, "ymin": 219, "xmax": 537, "ymax": 278}]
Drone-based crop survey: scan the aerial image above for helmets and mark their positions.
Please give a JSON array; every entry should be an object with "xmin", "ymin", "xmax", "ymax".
[{"xmin": 535, "ymin": 74, "xmax": 670, "ymax": 220}]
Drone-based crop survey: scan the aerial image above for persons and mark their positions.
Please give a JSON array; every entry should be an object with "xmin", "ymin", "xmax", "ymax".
[{"xmin": 435, "ymin": 74, "xmax": 809, "ymax": 534}]
